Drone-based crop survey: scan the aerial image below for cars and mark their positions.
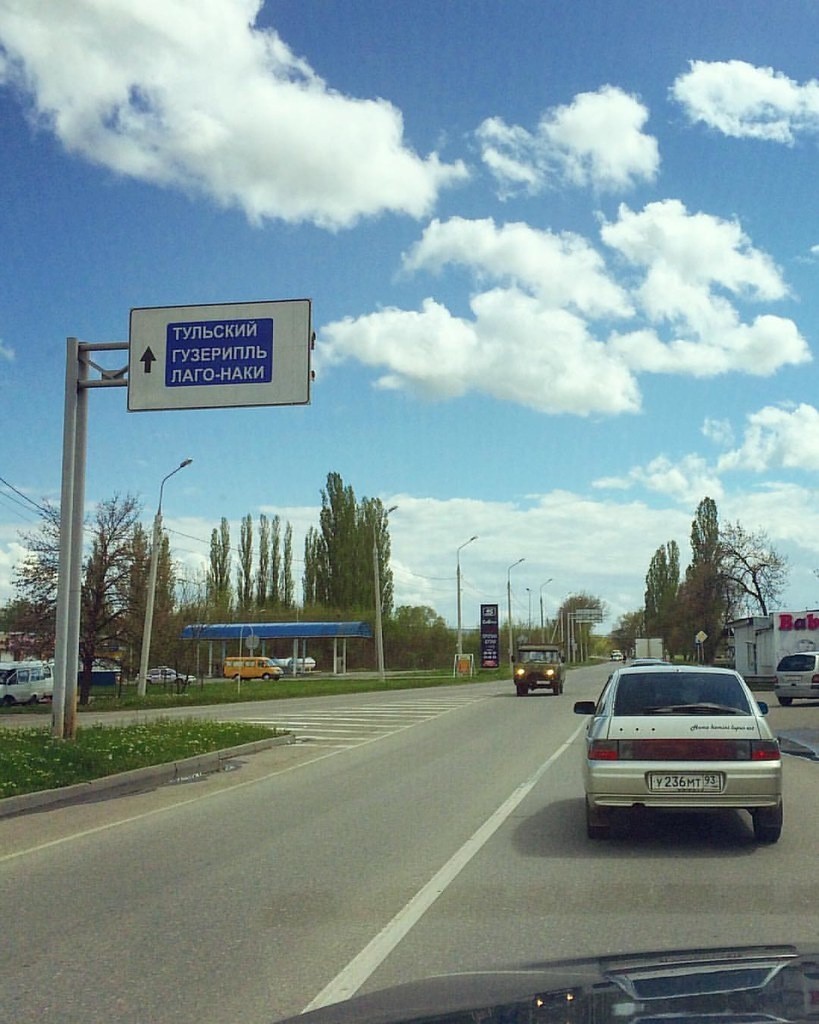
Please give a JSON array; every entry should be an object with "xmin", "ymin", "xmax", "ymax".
[
  {"xmin": 135, "ymin": 666, "xmax": 197, "ymax": 684},
  {"xmin": 610, "ymin": 649, "xmax": 624, "ymax": 661},
  {"xmin": 573, "ymin": 663, "xmax": 785, "ymax": 844},
  {"xmin": 631, "ymin": 659, "xmax": 663, "ymax": 667}
]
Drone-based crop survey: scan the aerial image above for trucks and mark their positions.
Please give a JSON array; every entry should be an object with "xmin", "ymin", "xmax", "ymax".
[{"xmin": 635, "ymin": 638, "xmax": 663, "ymax": 662}]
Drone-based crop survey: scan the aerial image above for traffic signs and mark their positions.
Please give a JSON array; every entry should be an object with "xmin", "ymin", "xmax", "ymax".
[{"xmin": 126, "ymin": 296, "xmax": 313, "ymax": 413}]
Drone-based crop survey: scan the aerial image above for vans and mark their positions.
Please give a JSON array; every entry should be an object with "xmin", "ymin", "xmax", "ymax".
[
  {"xmin": 224, "ymin": 657, "xmax": 284, "ymax": 681},
  {"xmin": 774, "ymin": 650, "xmax": 819, "ymax": 707},
  {"xmin": 0, "ymin": 658, "xmax": 54, "ymax": 706},
  {"xmin": 511, "ymin": 644, "xmax": 566, "ymax": 696}
]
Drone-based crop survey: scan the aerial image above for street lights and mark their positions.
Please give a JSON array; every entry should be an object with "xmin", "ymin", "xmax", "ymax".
[
  {"xmin": 456, "ymin": 535, "xmax": 478, "ymax": 653},
  {"xmin": 527, "ymin": 587, "xmax": 532, "ymax": 644},
  {"xmin": 507, "ymin": 557, "xmax": 526, "ymax": 660},
  {"xmin": 138, "ymin": 458, "xmax": 195, "ymax": 696},
  {"xmin": 539, "ymin": 577, "xmax": 553, "ymax": 644},
  {"xmin": 372, "ymin": 504, "xmax": 398, "ymax": 682}
]
